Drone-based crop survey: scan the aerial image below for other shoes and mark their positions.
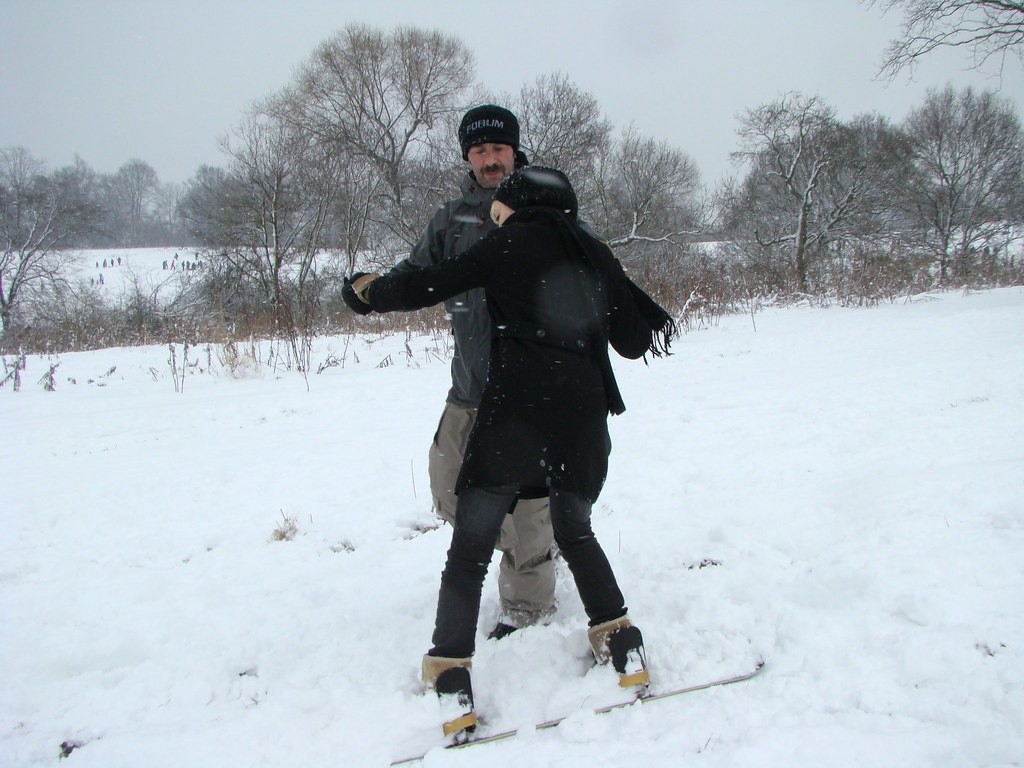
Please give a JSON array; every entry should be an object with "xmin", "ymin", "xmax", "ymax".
[
  {"xmin": 488, "ymin": 623, "xmax": 518, "ymax": 640},
  {"xmin": 421, "ymin": 654, "xmax": 476, "ymax": 734},
  {"xmin": 587, "ymin": 615, "xmax": 649, "ymax": 687}
]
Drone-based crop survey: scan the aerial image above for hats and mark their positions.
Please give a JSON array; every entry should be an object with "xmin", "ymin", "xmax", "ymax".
[
  {"xmin": 459, "ymin": 105, "xmax": 519, "ymax": 161},
  {"xmin": 493, "ymin": 166, "xmax": 578, "ymax": 218}
]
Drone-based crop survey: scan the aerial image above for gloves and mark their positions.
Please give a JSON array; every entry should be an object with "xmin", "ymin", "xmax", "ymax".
[{"xmin": 342, "ymin": 272, "xmax": 372, "ymax": 315}]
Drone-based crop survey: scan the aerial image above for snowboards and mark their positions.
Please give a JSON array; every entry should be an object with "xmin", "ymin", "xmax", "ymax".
[{"xmin": 390, "ymin": 651, "xmax": 764, "ymax": 768}]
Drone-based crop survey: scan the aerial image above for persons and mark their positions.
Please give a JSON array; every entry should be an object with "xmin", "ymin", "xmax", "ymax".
[
  {"xmin": 350, "ymin": 166, "xmax": 654, "ymax": 741},
  {"xmin": 340, "ymin": 103, "xmax": 613, "ymax": 643}
]
